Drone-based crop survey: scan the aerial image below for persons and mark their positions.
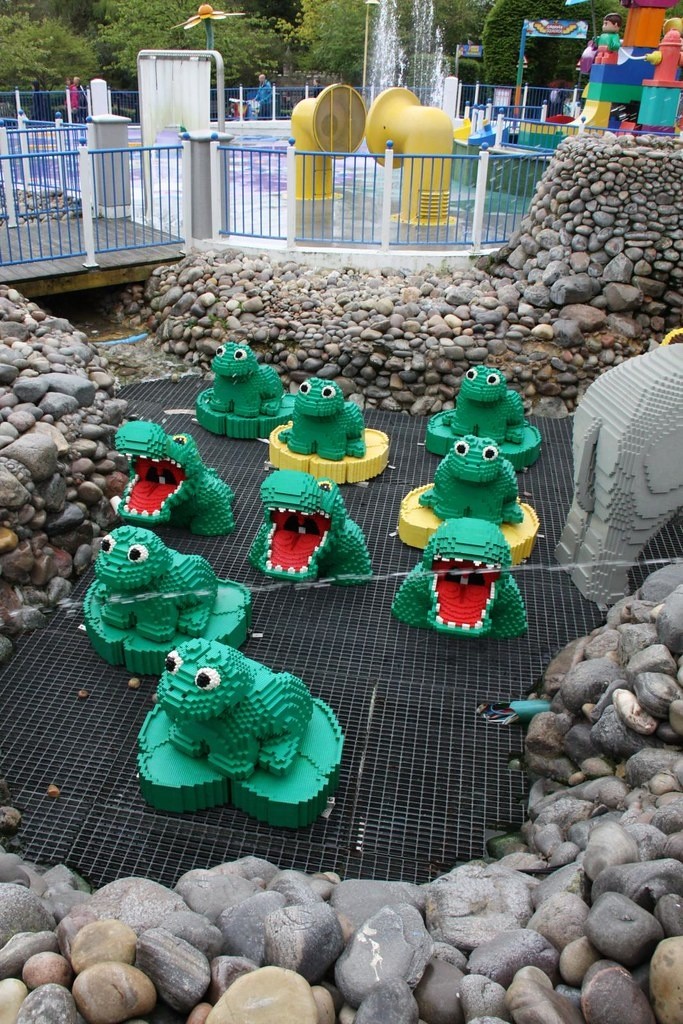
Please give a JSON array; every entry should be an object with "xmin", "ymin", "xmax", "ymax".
[
  {"xmin": 64, "ymin": 76, "xmax": 87, "ymax": 123},
  {"xmin": 593, "ymin": 13, "xmax": 623, "ymax": 65},
  {"xmin": 253, "ymin": 74, "xmax": 273, "ymax": 117},
  {"xmin": 313, "ymin": 78, "xmax": 324, "ymax": 98},
  {"xmin": 31, "ymin": 80, "xmax": 46, "ymax": 120}
]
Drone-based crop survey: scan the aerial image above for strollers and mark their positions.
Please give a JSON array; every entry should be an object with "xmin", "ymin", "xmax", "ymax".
[{"xmin": 228, "ymin": 99, "xmax": 261, "ymax": 120}]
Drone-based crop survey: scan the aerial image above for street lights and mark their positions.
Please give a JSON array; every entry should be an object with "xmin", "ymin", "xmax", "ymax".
[{"xmin": 362, "ymin": 0, "xmax": 381, "ymax": 100}]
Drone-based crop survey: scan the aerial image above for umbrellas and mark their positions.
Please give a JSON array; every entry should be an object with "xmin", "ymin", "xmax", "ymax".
[{"xmin": 565, "ymin": 0, "xmax": 597, "ymax": 37}]
